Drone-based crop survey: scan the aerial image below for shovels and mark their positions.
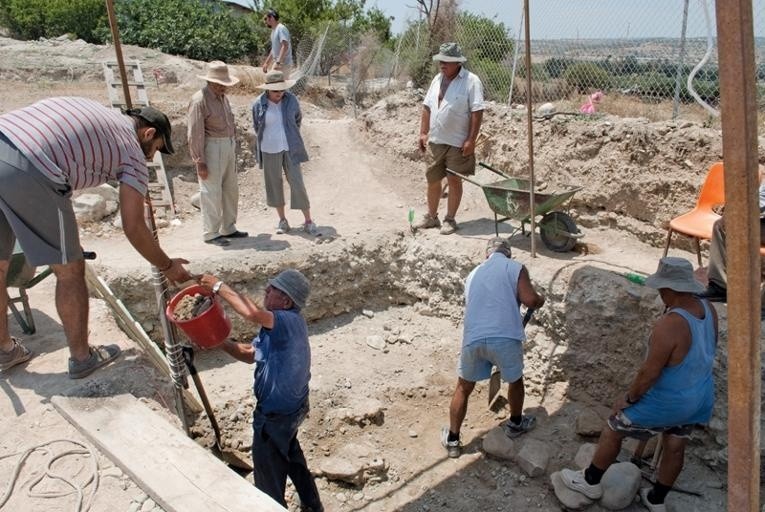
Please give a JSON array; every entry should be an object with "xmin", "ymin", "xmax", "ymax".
[
  {"xmin": 488, "ymin": 289, "xmax": 544, "ymax": 405},
  {"xmin": 181, "ymin": 343, "xmax": 254, "ymax": 470}
]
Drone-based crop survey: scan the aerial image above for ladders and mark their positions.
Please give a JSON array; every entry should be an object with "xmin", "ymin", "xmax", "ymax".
[{"xmin": 101, "ymin": 58, "xmax": 176, "ymax": 220}]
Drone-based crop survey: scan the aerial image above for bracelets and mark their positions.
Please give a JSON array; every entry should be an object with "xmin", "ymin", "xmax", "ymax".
[
  {"xmin": 160, "ymin": 259, "xmax": 172, "ymax": 271},
  {"xmin": 276, "ymin": 60, "xmax": 280, "ymax": 65}
]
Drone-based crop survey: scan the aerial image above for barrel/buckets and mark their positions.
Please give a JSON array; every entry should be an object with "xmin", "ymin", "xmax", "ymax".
[{"xmin": 160, "ymin": 271, "xmax": 232, "ymax": 348}]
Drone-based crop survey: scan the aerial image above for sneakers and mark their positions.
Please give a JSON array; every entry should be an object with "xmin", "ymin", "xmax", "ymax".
[
  {"xmin": 560, "ymin": 468, "xmax": 603, "ymax": 500},
  {"xmin": 441, "ymin": 426, "xmax": 460, "ymax": 457},
  {"xmin": 441, "ymin": 218, "xmax": 460, "ymax": 234},
  {"xmin": 302, "ymin": 223, "xmax": 322, "ymax": 236},
  {"xmin": 68, "ymin": 343, "xmax": 121, "ymax": 380},
  {"xmin": 414, "ymin": 213, "xmax": 440, "ymax": 228},
  {"xmin": 696, "ymin": 282, "xmax": 727, "ymax": 307},
  {"xmin": 639, "ymin": 487, "xmax": 666, "ymax": 512},
  {"xmin": 275, "ymin": 218, "xmax": 290, "ymax": 234},
  {"xmin": 0, "ymin": 336, "xmax": 34, "ymax": 371},
  {"xmin": 505, "ymin": 414, "xmax": 537, "ymax": 437}
]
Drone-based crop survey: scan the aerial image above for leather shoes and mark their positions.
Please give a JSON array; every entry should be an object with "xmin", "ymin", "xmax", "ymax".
[
  {"xmin": 204, "ymin": 236, "xmax": 231, "ymax": 247},
  {"xmin": 221, "ymin": 230, "xmax": 248, "ymax": 238}
]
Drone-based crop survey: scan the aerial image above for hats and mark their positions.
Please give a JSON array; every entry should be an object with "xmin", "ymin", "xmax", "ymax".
[
  {"xmin": 126, "ymin": 106, "xmax": 174, "ymax": 157},
  {"xmin": 643, "ymin": 257, "xmax": 708, "ymax": 295},
  {"xmin": 195, "ymin": 59, "xmax": 239, "ymax": 87},
  {"xmin": 268, "ymin": 268, "xmax": 312, "ymax": 310},
  {"xmin": 255, "ymin": 71, "xmax": 297, "ymax": 91},
  {"xmin": 485, "ymin": 235, "xmax": 513, "ymax": 252},
  {"xmin": 431, "ymin": 41, "xmax": 470, "ymax": 63}
]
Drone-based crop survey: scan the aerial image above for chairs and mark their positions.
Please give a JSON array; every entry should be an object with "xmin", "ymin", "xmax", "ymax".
[{"xmin": 662, "ymin": 162, "xmax": 764, "ymax": 268}]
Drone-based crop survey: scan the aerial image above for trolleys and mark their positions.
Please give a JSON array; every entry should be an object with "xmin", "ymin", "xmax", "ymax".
[
  {"xmin": 5, "ymin": 236, "xmax": 96, "ymax": 335},
  {"xmin": 446, "ymin": 161, "xmax": 585, "ymax": 252}
]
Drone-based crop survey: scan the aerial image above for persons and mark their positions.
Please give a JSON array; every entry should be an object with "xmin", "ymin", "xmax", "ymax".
[
  {"xmin": 0, "ymin": 96, "xmax": 192, "ymax": 380},
  {"xmin": 692, "ymin": 177, "xmax": 765, "ymax": 322},
  {"xmin": 559, "ymin": 257, "xmax": 719, "ymax": 512},
  {"xmin": 263, "ymin": 7, "xmax": 294, "ymax": 80},
  {"xmin": 187, "ymin": 60, "xmax": 248, "ymax": 247},
  {"xmin": 414, "ymin": 41, "xmax": 487, "ymax": 235},
  {"xmin": 192, "ymin": 268, "xmax": 325, "ymax": 512},
  {"xmin": 440, "ymin": 237, "xmax": 546, "ymax": 457},
  {"xmin": 252, "ymin": 69, "xmax": 323, "ymax": 237}
]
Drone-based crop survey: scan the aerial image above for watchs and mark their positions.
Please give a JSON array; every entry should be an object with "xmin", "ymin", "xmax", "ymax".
[
  {"xmin": 624, "ymin": 393, "xmax": 639, "ymax": 405},
  {"xmin": 212, "ymin": 280, "xmax": 224, "ymax": 294}
]
